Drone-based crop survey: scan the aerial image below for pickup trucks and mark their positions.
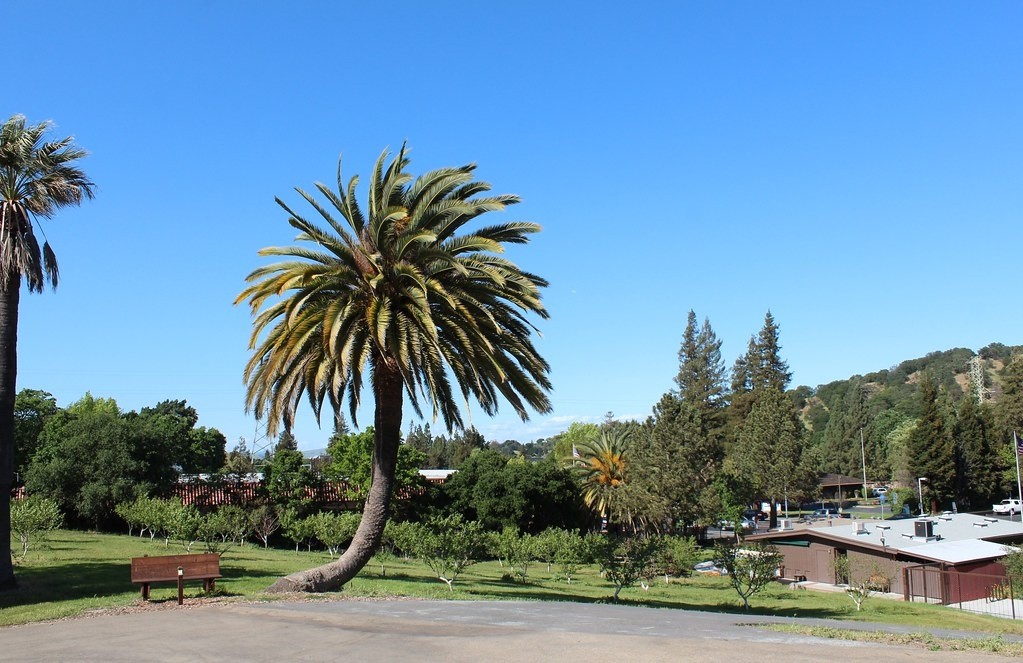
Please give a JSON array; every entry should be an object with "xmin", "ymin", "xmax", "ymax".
[
  {"xmin": 803, "ymin": 509, "xmax": 850, "ymax": 520},
  {"xmin": 992, "ymin": 498, "xmax": 1023, "ymax": 516}
]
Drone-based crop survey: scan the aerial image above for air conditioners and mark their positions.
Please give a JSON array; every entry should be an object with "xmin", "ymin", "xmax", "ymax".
[{"xmin": 781, "ymin": 520, "xmax": 791, "ymax": 529}]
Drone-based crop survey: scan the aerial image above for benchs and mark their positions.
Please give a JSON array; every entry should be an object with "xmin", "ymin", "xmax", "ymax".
[
  {"xmin": 131, "ymin": 551, "xmax": 223, "ymax": 600},
  {"xmin": 866, "ymin": 573, "xmax": 890, "ymax": 594}
]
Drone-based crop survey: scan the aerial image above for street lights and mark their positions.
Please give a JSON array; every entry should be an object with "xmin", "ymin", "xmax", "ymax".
[{"xmin": 918, "ymin": 477, "xmax": 928, "ymax": 514}]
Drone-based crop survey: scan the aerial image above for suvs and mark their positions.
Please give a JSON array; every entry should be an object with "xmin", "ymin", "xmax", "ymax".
[
  {"xmin": 810, "ymin": 503, "xmax": 837, "ymax": 514},
  {"xmin": 872, "ymin": 488, "xmax": 888, "ymax": 497}
]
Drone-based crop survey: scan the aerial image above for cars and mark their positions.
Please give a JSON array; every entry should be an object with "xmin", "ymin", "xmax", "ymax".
[
  {"xmin": 718, "ymin": 514, "xmax": 759, "ymax": 530},
  {"xmin": 177, "ymin": 472, "xmax": 265, "ymax": 483},
  {"xmin": 740, "ymin": 510, "xmax": 768, "ymax": 521}
]
